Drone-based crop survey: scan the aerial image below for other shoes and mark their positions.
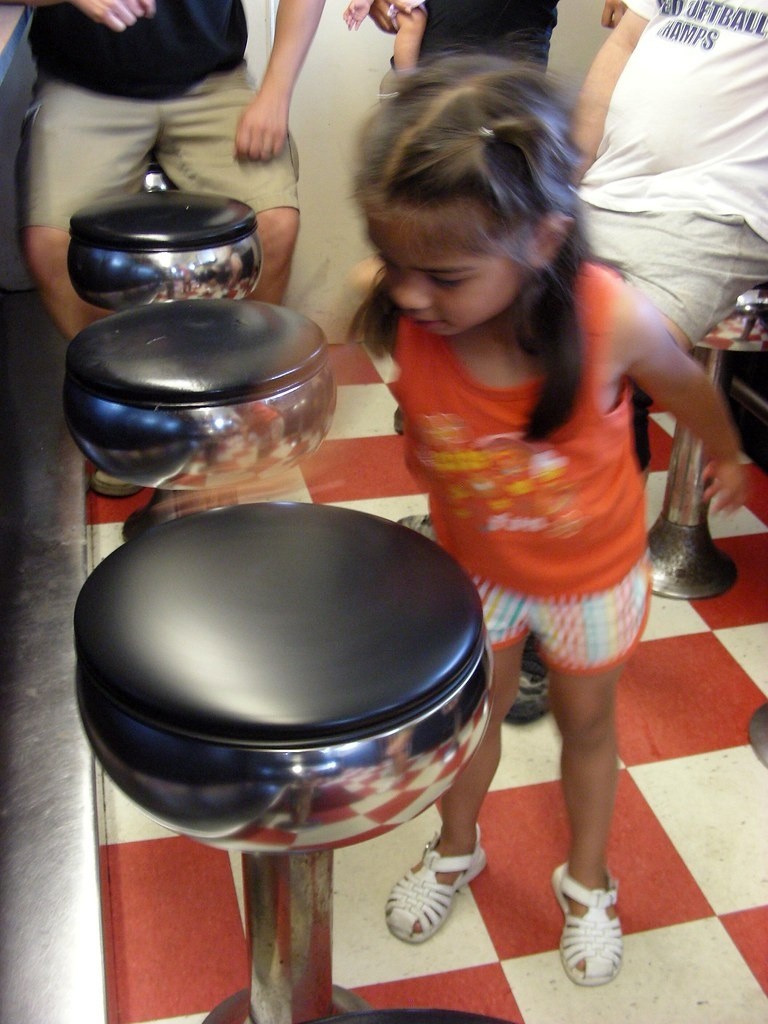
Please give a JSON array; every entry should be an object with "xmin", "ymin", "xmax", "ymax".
[{"xmin": 501, "ymin": 636, "xmax": 558, "ymax": 724}]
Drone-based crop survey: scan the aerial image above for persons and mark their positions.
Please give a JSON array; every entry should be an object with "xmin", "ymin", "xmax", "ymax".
[
  {"xmin": 344, "ymin": 52, "xmax": 751, "ymax": 987},
  {"xmin": 2, "ymin": 0, "xmax": 768, "ymax": 723}
]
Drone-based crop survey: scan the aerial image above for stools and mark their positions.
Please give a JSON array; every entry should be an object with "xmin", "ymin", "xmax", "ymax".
[
  {"xmin": 71, "ymin": 190, "xmax": 511, "ymax": 1024},
  {"xmin": 643, "ymin": 289, "xmax": 768, "ymax": 602}
]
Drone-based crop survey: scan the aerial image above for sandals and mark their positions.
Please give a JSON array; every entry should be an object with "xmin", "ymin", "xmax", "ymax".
[
  {"xmin": 385, "ymin": 817, "xmax": 486, "ymax": 943},
  {"xmin": 553, "ymin": 860, "xmax": 622, "ymax": 987}
]
